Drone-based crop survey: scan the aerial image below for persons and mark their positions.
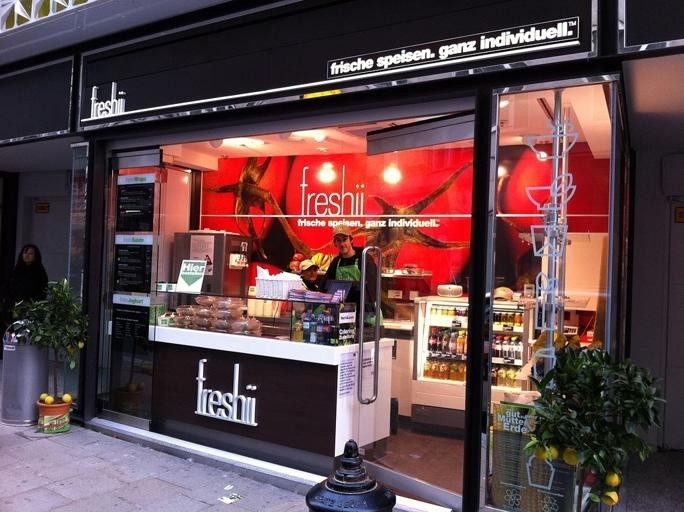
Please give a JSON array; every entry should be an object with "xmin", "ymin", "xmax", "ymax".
[
  {"xmin": 326, "ymin": 224, "xmax": 384, "ymax": 327},
  {"xmin": 299, "ymin": 258, "xmax": 321, "ymax": 294},
  {"xmin": 11, "ymin": 243, "xmax": 49, "ymax": 303}
]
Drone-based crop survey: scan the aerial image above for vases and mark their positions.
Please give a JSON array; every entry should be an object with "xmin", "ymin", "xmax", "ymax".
[{"xmin": 119, "ymin": 386, "xmax": 143, "ymax": 407}]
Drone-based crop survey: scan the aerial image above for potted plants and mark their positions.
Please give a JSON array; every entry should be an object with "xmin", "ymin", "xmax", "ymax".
[{"xmin": 14, "ymin": 280, "xmax": 89, "ymax": 433}]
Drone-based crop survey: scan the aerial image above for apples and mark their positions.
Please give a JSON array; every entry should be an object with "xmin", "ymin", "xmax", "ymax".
[{"xmin": 289, "ymin": 253, "xmax": 304, "ymax": 270}]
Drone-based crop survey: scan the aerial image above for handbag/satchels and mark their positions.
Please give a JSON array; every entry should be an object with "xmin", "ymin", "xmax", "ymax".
[{"xmin": 437, "ymin": 285, "xmax": 463, "ymax": 298}]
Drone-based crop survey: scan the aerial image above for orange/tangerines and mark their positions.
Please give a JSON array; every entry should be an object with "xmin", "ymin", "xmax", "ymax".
[
  {"xmin": 63, "ymin": 394, "xmax": 71, "ymax": 402},
  {"xmin": 40, "ymin": 394, "xmax": 54, "ymax": 404},
  {"xmin": 68, "ymin": 345, "xmax": 75, "ymax": 352},
  {"xmin": 605, "ymin": 472, "xmax": 620, "ymax": 486},
  {"xmin": 534, "ymin": 446, "xmax": 558, "ymax": 460},
  {"xmin": 602, "ymin": 492, "xmax": 619, "ymax": 506},
  {"xmin": 564, "ymin": 448, "xmax": 579, "ymax": 465}
]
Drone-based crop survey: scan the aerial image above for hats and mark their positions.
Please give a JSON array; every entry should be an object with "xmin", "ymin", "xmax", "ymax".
[
  {"xmin": 493, "ymin": 287, "xmax": 513, "ymax": 300},
  {"xmin": 333, "ymin": 224, "xmax": 352, "ymax": 238},
  {"xmin": 300, "ymin": 259, "xmax": 319, "ymax": 273}
]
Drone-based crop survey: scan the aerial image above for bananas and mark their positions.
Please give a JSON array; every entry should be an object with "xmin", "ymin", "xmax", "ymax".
[{"xmin": 311, "ymin": 253, "xmax": 333, "ymax": 271}]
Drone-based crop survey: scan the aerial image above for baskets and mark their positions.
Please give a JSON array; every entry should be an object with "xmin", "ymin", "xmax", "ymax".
[{"xmin": 255, "ymin": 277, "xmax": 302, "ymax": 300}]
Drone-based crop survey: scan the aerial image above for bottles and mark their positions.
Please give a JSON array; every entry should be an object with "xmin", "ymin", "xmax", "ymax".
[
  {"xmin": 289, "ymin": 306, "xmax": 341, "ymax": 347},
  {"xmin": 420, "ymin": 304, "xmax": 526, "ymax": 391}
]
